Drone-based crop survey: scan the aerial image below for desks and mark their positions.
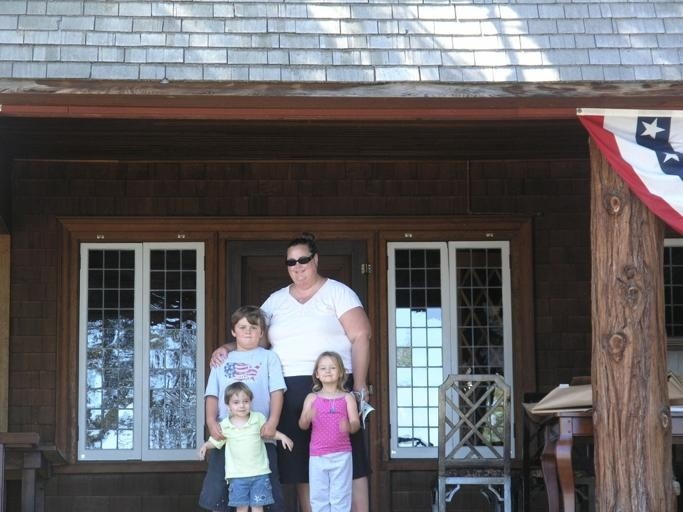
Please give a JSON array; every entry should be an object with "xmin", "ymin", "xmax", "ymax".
[
  {"xmin": 530, "ymin": 411, "xmax": 683, "ymax": 512},
  {"xmin": 0, "ymin": 431, "xmax": 64, "ymax": 512}
]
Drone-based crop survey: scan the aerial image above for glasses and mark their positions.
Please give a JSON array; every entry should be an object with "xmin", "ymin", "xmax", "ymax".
[{"xmin": 285, "ymin": 251, "xmax": 316, "ymax": 267}]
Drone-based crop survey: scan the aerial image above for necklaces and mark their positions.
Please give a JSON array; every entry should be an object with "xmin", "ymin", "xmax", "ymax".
[
  {"xmin": 292, "ymin": 287, "xmax": 316, "ymax": 303},
  {"xmin": 322, "ymin": 387, "xmax": 339, "ymax": 414}
]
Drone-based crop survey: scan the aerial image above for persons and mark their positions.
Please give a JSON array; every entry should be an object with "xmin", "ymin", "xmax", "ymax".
[
  {"xmin": 197, "ymin": 306, "xmax": 288, "ymax": 512},
  {"xmin": 208, "ymin": 233, "xmax": 372, "ymax": 511},
  {"xmin": 298, "ymin": 352, "xmax": 360, "ymax": 511},
  {"xmin": 199, "ymin": 382, "xmax": 295, "ymax": 512}
]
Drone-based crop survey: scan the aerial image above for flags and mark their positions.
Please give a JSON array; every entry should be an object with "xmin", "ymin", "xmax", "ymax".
[{"xmin": 576, "ymin": 107, "xmax": 682, "ymax": 238}]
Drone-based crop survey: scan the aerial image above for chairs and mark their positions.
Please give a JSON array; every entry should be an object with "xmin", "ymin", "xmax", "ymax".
[{"xmin": 433, "ymin": 373, "xmax": 512, "ymax": 512}]
